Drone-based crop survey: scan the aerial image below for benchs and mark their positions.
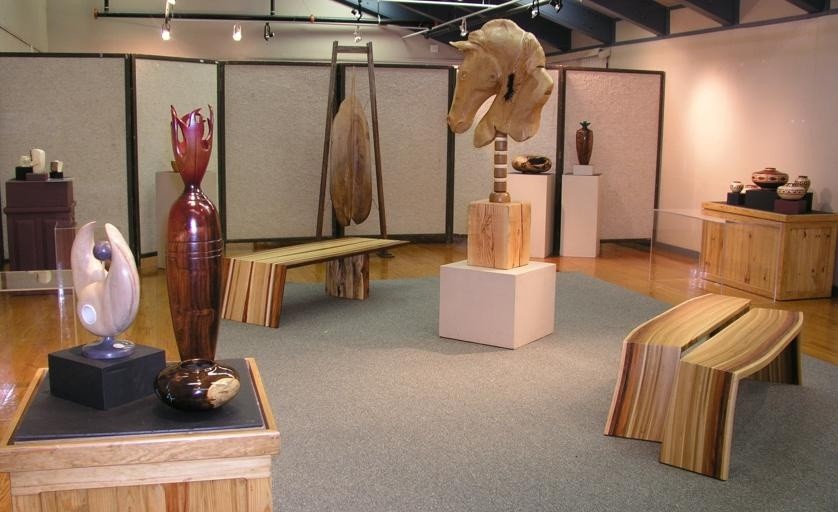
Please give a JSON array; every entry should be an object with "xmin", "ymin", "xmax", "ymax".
[
  {"xmin": 659, "ymin": 308, "xmax": 805, "ymax": 481},
  {"xmin": 223, "ymin": 238, "xmax": 410, "ymax": 327},
  {"xmin": 604, "ymin": 294, "xmax": 752, "ymax": 442}
]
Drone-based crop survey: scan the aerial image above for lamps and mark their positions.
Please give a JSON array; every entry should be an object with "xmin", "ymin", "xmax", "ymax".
[
  {"xmin": 95, "ymin": 8, "xmax": 433, "ymax": 41},
  {"xmin": 425, "ymin": 0, "xmax": 562, "ymax": 37}
]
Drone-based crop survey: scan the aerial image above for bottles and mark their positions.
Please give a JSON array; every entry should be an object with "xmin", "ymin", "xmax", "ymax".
[{"xmin": 575, "ymin": 126, "xmax": 595, "ymax": 165}]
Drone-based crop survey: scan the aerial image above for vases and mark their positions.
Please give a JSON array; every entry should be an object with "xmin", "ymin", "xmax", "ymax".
[
  {"xmin": 576, "ymin": 122, "xmax": 593, "ymax": 165},
  {"xmin": 155, "ymin": 358, "xmax": 240, "ymax": 414},
  {"xmin": 165, "ymin": 103, "xmax": 224, "ymax": 361},
  {"xmin": 730, "ymin": 168, "xmax": 810, "ymax": 201}
]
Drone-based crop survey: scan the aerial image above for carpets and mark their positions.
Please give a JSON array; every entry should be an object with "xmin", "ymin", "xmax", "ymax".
[{"xmin": 215, "ymin": 271, "xmax": 838, "ymax": 512}]
